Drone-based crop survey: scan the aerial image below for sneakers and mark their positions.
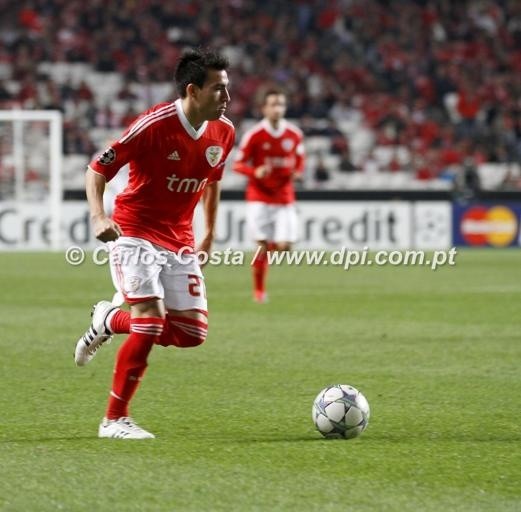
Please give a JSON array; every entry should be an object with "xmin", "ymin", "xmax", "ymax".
[
  {"xmin": 253, "ymin": 285, "xmax": 265, "ymax": 306},
  {"xmin": 73, "ymin": 299, "xmax": 119, "ymax": 368},
  {"xmin": 98, "ymin": 416, "xmax": 156, "ymax": 440}
]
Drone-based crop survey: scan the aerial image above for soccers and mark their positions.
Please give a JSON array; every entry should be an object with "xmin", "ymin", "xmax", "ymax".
[{"xmin": 312, "ymin": 384, "xmax": 369, "ymax": 439}]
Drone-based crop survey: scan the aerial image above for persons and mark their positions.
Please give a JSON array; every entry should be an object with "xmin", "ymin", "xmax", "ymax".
[
  {"xmin": 74, "ymin": 44, "xmax": 235, "ymax": 439},
  {"xmin": 232, "ymin": 89, "xmax": 307, "ymax": 303},
  {"xmin": 1, "ymin": 0, "xmax": 520, "ymax": 190}
]
